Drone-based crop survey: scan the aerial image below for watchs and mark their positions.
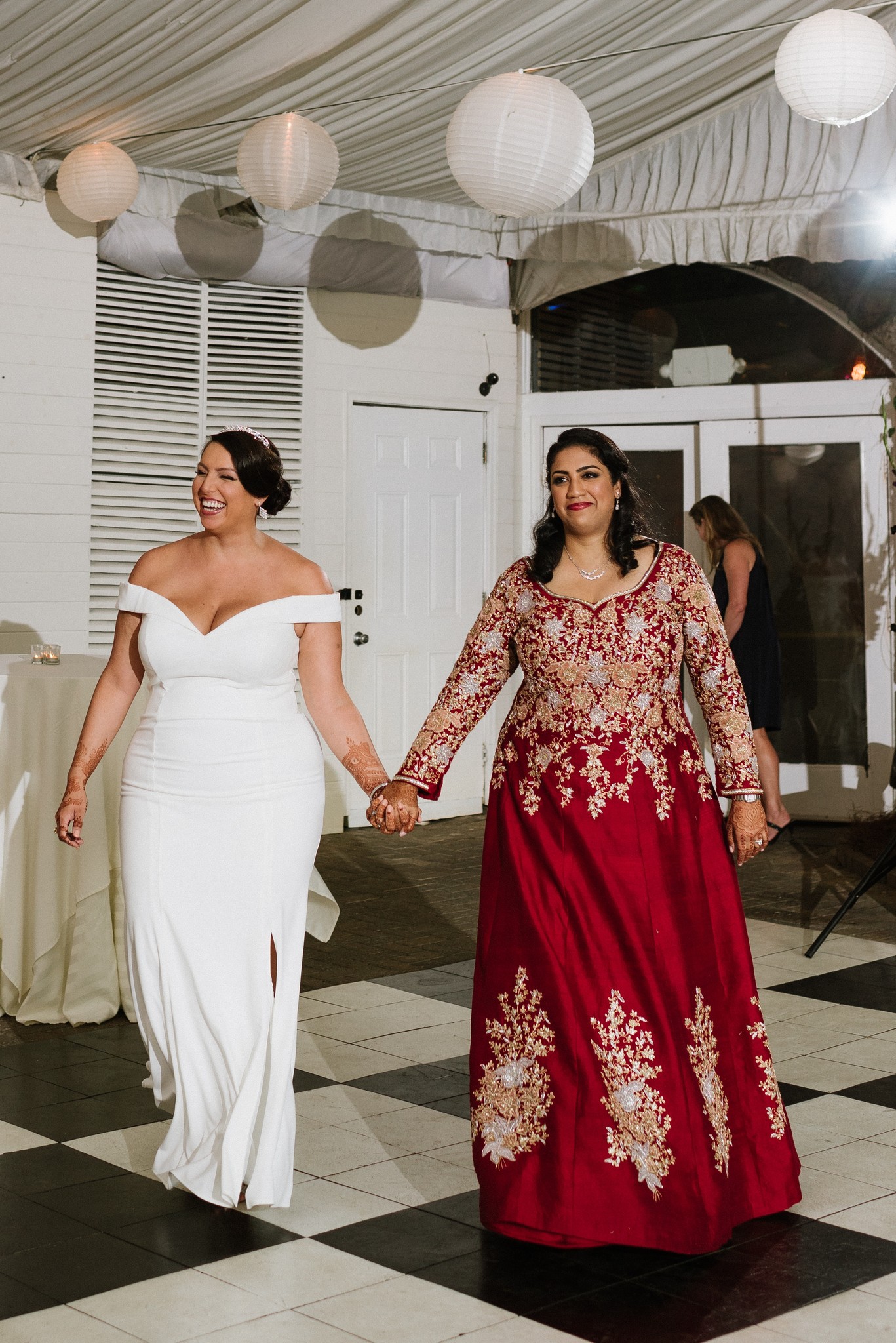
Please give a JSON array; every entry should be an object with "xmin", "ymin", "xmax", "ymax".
[{"xmin": 731, "ymin": 794, "xmax": 761, "ymax": 803}]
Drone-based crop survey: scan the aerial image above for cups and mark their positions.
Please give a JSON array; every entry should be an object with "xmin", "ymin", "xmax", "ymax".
[
  {"xmin": 45, "ymin": 644, "xmax": 58, "ymax": 646},
  {"xmin": 29, "ymin": 644, "xmax": 45, "ymax": 664},
  {"xmin": 44, "ymin": 645, "xmax": 61, "ymax": 665}
]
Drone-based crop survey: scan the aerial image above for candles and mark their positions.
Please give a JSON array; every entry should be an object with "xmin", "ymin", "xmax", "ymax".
[
  {"xmin": 33, "ymin": 655, "xmax": 42, "ymax": 662},
  {"xmin": 46, "ymin": 654, "xmax": 59, "ymax": 662}
]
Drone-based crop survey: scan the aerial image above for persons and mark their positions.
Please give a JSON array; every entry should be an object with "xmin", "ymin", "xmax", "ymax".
[
  {"xmin": 364, "ymin": 427, "xmax": 804, "ymax": 1258},
  {"xmin": 53, "ymin": 425, "xmax": 426, "ymax": 1213},
  {"xmin": 789, "ymin": 528, "xmax": 865, "ymax": 766},
  {"xmin": 688, "ymin": 494, "xmax": 795, "ymax": 845}
]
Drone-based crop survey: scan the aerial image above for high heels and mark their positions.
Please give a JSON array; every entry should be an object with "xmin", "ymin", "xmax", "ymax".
[{"xmin": 766, "ymin": 818, "xmax": 797, "ymax": 844}]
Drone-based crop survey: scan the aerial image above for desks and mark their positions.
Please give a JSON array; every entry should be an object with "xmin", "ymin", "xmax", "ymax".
[{"xmin": 2, "ymin": 652, "xmax": 149, "ymax": 1033}]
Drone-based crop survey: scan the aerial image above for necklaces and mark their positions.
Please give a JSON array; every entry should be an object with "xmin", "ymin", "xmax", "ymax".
[
  {"xmin": 369, "ymin": 782, "xmax": 390, "ymax": 802},
  {"xmin": 564, "ymin": 543, "xmax": 612, "ymax": 581}
]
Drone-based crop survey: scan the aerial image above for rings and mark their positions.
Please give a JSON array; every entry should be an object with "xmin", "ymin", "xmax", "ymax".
[
  {"xmin": 371, "ymin": 810, "xmax": 377, "ymax": 816},
  {"xmin": 377, "ymin": 824, "xmax": 381, "ymax": 827},
  {"xmin": 754, "ymin": 838, "xmax": 763, "ymax": 845},
  {"xmin": 53, "ymin": 826, "xmax": 58, "ymax": 834}
]
